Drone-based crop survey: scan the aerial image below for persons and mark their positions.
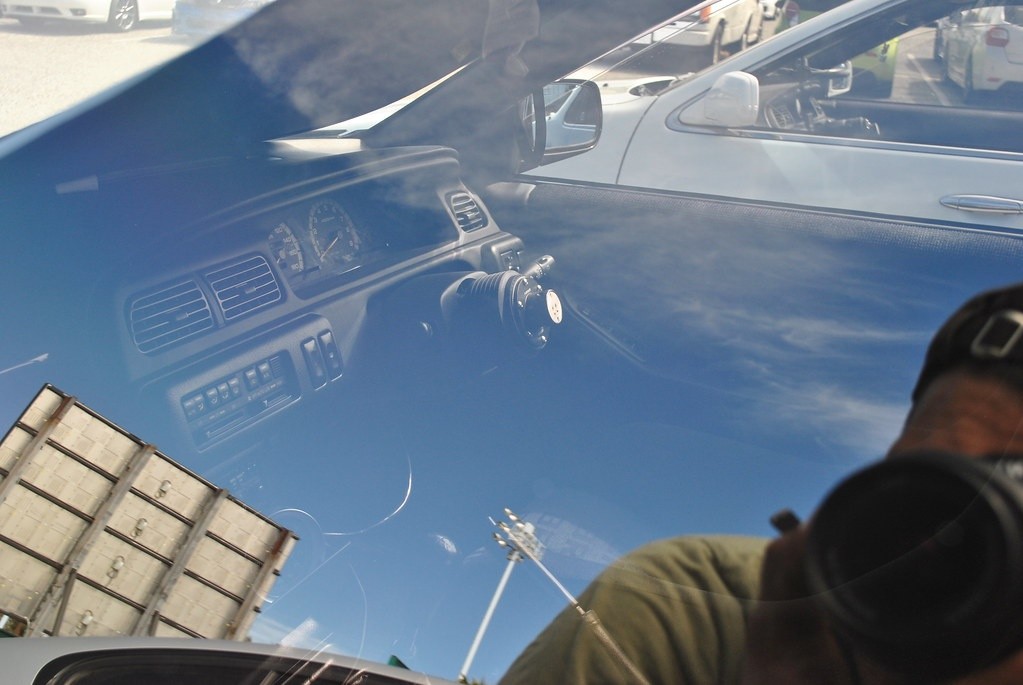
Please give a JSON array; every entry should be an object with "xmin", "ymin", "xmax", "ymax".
[{"xmin": 497, "ymin": 286, "xmax": 1023, "ymax": 685}]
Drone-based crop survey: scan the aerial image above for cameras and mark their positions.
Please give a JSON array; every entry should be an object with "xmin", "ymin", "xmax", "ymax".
[{"xmin": 808, "ymin": 455, "xmax": 1023, "ymax": 682}]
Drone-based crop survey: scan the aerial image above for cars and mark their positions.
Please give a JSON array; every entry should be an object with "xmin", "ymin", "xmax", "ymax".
[
  {"xmin": 331, "ymin": 1, "xmax": 1022, "ymax": 230},
  {"xmin": 774, "ymin": 0, "xmax": 898, "ymax": 99},
  {"xmin": 626, "ymin": 1, "xmax": 764, "ymax": 69},
  {"xmin": 1, "ymin": 0, "xmax": 178, "ymax": 35},
  {"xmin": 930, "ymin": 6, "xmax": 1023, "ymax": 104},
  {"xmin": 762, "ymin": 1, "xmax": 785, "ymax": 20}
]
